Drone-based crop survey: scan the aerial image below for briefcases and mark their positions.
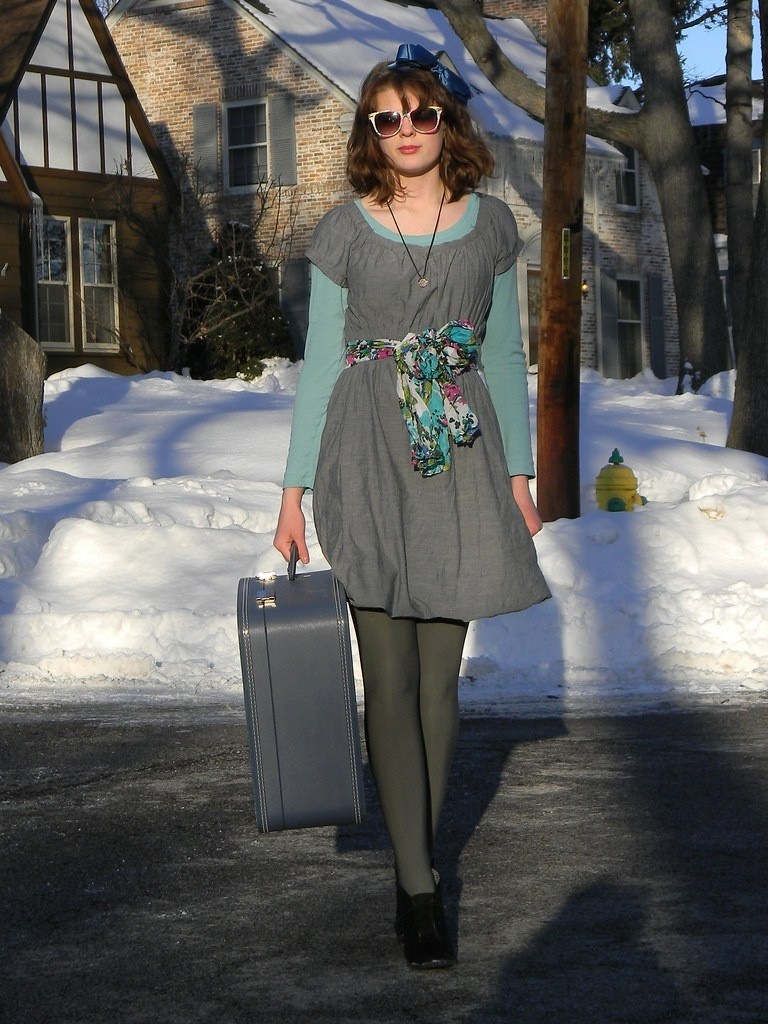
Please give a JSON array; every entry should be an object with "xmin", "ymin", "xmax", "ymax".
[{"xmin": 237, "ymin": 541, "xmax": 368, "ymax": 834}]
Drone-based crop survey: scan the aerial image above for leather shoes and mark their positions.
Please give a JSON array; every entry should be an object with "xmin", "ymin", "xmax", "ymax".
[{"xmin": 394, "ymin": 857, "xmax": 451, "ymax": 970}]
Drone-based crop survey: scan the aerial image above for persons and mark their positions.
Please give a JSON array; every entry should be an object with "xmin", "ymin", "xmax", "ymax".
[{"xmin": 274, "ymin": 43, "xmax": 543, "ymax": 968}]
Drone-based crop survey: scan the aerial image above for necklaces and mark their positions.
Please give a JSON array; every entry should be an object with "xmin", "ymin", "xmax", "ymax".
[{"xmin": 386, "ymin": 184, "xmax": 446, "ymax": 288}]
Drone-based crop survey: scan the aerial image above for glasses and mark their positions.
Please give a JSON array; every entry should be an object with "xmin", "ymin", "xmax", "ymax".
[{"xmin": 368, "ymin": 107, "xmax": 443, "ymax": 137}]
